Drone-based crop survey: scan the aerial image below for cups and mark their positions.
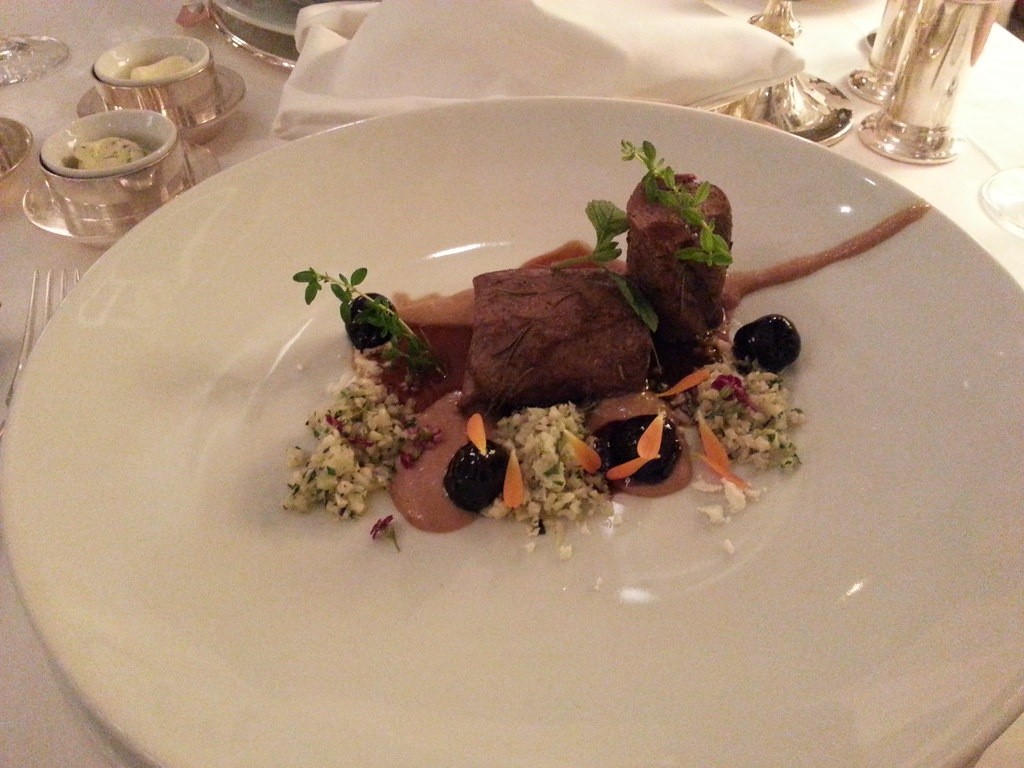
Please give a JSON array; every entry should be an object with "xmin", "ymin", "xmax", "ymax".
[
  {"xmin": 91, "ymin": 51, "xmax": 224, "ymax": 125},
  {"xmin": 37, "ymin": 131, "xmax": 195, "ymax": 236}
]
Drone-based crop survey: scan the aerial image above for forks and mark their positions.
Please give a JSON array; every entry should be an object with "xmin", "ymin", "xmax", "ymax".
[{"xmin": 0, "ymin": 267, "xmax": 81, "ymax": 434}]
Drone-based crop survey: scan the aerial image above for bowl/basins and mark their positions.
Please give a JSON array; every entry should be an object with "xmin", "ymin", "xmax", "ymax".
[
  {"xmin": 93, "ymin": 35, "xmax": 210, "ymax": 87},
  {"xmin": 41, "ymin": 108, "xmax": 177, "ymax": 178}
]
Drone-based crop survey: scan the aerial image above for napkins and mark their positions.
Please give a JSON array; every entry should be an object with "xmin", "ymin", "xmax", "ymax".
[{"xmin": 268, "ymin": 0, "xmax": 810, "ymax": 140}]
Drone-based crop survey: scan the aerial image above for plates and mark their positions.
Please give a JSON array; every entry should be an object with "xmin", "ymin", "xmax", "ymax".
[
  {"xmin": 75, "ymin": 63, "xmax": 246, "ymax": 146},
  {"xmin": 21, "ymin": 140, "xmax": 221, "ymax": 247},
  {"xmin": 0, "ymin": 98, "xmax": 1023, "ymax": 768}
]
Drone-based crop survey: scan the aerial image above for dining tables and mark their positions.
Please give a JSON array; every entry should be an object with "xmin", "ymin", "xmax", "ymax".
[{"xmin": 0, "ymin": 0, "xmax": 1024, "ymax": 768}]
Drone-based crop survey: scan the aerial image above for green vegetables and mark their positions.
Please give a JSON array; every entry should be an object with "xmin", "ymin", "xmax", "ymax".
[{"xmin": 291, "ymin": 135, "xmax": 734, "ymax": 371}]
